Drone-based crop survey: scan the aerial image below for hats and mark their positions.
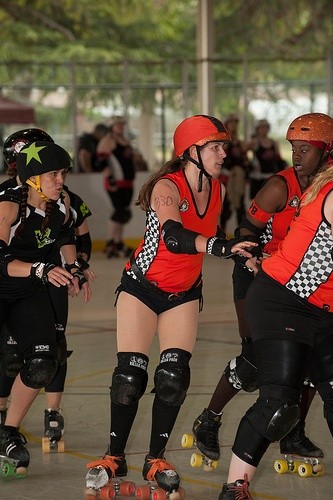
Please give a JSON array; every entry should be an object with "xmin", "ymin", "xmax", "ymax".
[
  {"xmin": 255, "ymin": 120, "xmax": 269, "ymax": 130},
  {"xmin": 221, "ymin": 115, "xmax": 239, "ymax": 127}
]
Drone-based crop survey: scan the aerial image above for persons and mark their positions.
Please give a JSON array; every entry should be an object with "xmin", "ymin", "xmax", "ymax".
[
  {"xmin": 95, "ymin": 115, "xmax": 146, "ymax": 258},
  {"xmin": 0, "ymin": 128, "xmax": 96, "ymax": 478},
  {"xmin": 182, "ymin": 113, "xmax": 333, "ymax": 500},
  {"xmin": 74, "ymin": 122, "xmax": 109, "ymax": 175},
  {"xmin": 83, "ymin": 115, "xmax": 258, "ymax": 500}
]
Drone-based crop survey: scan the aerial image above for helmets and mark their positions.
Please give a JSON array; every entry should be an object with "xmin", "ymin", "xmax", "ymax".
[
  {"xmin": 16, "ymin": 141, "xmax": 71, "ymax": 185},
  {"xmin": 3, "ymin": 128, "xmax": 54, "ymax": 172},
  {"xmin": 173, "ymin": 114, "xmax": 232, "ymax": 159},
  {"xmin": 286, "ymin": 112, "xmax": 333, "ymax": 147},
  {"xmin": 107, "ymin": 115, "xmax": 126, "ymax": 130}
]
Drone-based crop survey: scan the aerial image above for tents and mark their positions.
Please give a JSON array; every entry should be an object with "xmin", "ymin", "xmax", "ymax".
[{"xmin": 0, "ymin": 96, "xmax": 35, "ymax": 131}]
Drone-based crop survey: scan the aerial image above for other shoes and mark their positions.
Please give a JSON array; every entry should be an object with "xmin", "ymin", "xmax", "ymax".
[
  {"xmin": 103, "ymin": 240, "xmax": 133, "ymax": 259},
  {"xmin": 218, "ymin": 472, "xmax": 253, "ymax": 500}
]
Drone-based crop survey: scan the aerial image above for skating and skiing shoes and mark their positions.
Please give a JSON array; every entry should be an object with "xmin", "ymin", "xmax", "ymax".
[
  {"xmin": 82, "ymin": 449, "xmax": 136, "ymax": 500},
  {"xmin": 0, "ymin": 425, "xmax": 31, "ymax": 479},
  {"xmin": 135, "ymin": 448, "xmax": 186, "ymax": 500},
  {"xmin": 182, "ymin": 407, "xmax": 224, "ymax": 471},
  {"xmin": 41, "ymin": 408, "xmax": 66, "ymax": 455},
  {"xmin": 273, "ymin": 419, "xmax": 326, "ymax": 477}
]
